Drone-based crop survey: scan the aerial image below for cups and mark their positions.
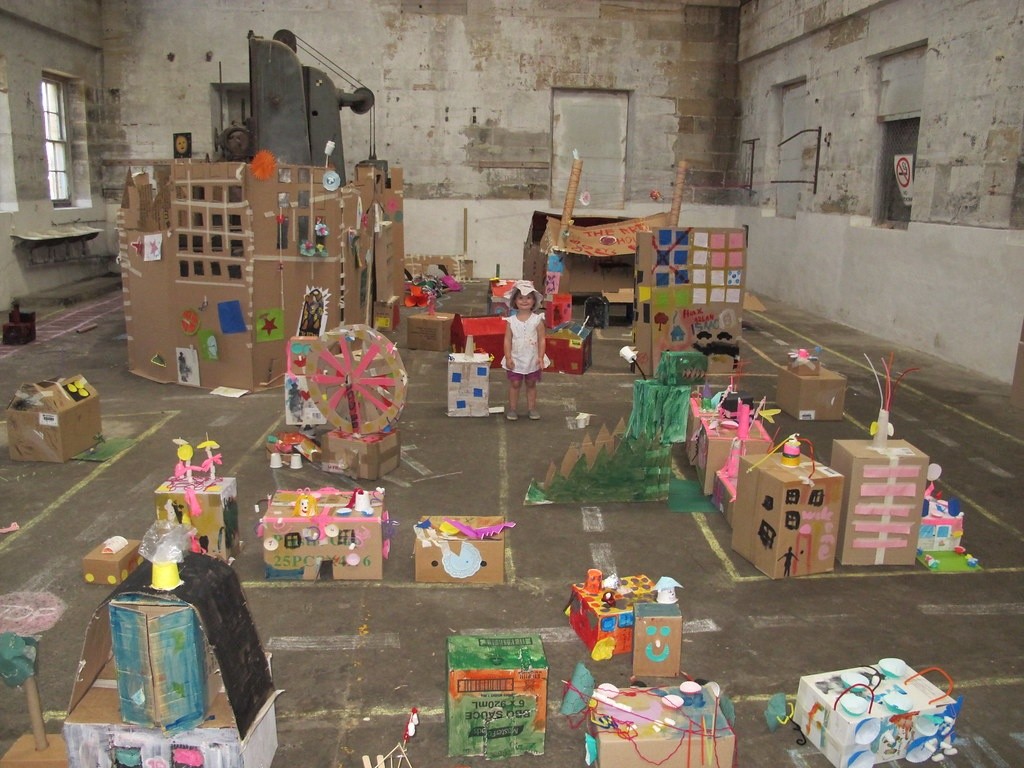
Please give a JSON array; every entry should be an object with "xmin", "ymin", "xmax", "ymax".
[
  {"xmin": 269, "ymin": 454, "xmax": 284, "ymax": 471},
  {"xmin": 658, "ymin": 582, "xmax": 677, "ymax": 606},
  {"xmin": 780, "ymin": 441, "xmax": 802, "ymax": 468},
  {"xmin": 299, "ymin": 494, "xmax": 316, "ymax": 517},
  {"xmin": 356, "ymin": 491, "xmax": 371, "ymax": 511},
  {"xmin": 576, "ymin": 418, "xmax": 586, "ymax": 429},
  {"xmin": 619, "ymin": 346, "xmax": 637, "ymax": 364},
  {"xmin": 150, "ymin": 558, "xmax": 181, "ymax": 591},
  {"xmin": 290, "ymin": 454, "xmax": 303, "ymax": 470},
  {"xmin": 586, "ymin": 415, "xmax": 590, "ymax": 426}
]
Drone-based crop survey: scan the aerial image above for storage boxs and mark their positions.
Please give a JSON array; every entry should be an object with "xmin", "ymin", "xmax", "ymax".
[{"xmin": 1, "ymin": 160, "xmax": 981, "ymax": 765}]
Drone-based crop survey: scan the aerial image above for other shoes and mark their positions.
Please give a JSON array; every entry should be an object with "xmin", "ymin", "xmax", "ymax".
[
  {"xmin": 528, "ymin": 408, "xmax": 541, "ymax": 420},
  {"xmin": 506, "ymin": 409, "xmax": 519, "ymax": 421}
]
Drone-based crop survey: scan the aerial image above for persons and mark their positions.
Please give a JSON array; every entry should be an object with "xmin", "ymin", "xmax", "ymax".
[{"xmin": 500, "ymin": 286, "xmax": 548, "ymax": 421}]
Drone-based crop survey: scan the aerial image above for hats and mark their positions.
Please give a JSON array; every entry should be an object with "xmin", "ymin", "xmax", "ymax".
[{"xmin": 503, "ymin": 279, "xmax": 544, "ymax": 312}]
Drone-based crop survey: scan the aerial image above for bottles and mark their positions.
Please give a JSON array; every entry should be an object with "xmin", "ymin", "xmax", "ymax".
[{"xmin": 702, "ymin": 381, "xmax": 712, "ymax": 411}]
